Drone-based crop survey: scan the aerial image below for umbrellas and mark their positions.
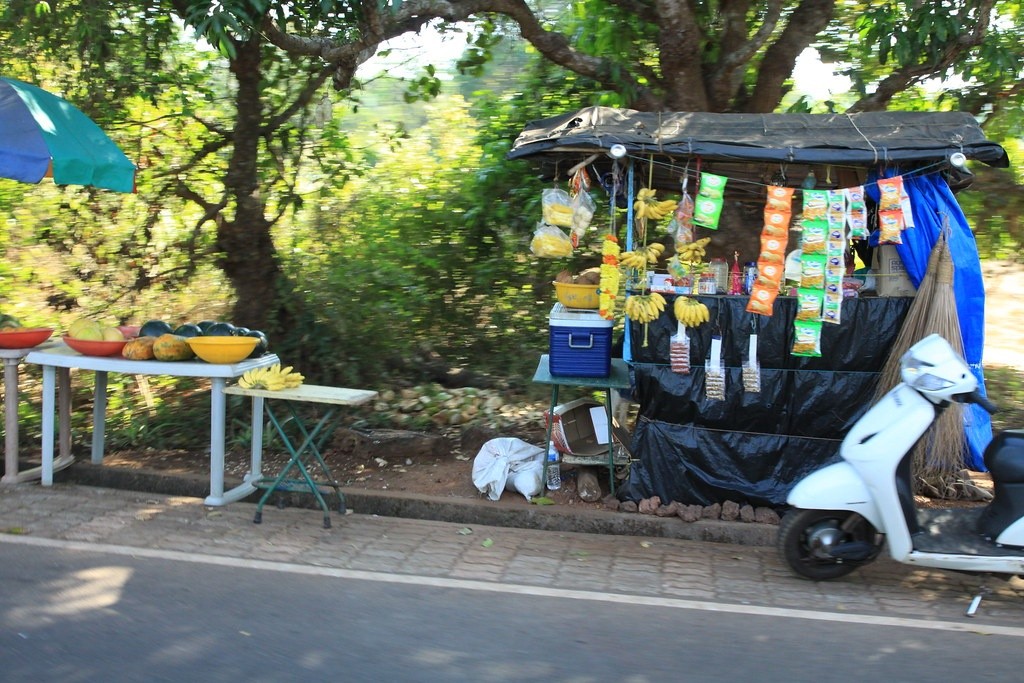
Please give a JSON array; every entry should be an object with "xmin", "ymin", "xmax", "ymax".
[{"xmin": 0, "ymin": 75, "xmax": 136, "ymax": 194}]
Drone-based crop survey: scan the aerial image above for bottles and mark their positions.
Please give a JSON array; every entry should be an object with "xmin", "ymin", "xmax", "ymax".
[
  {"xmin": 729, "ymin": 262, "xmax": 742, "ymax": 296},
  {"xmin": 708, "ymin": 258, "xmax": 728, "ymax": 293},
  {"xmin": 698, "ymin": 274, "xmax": 716, "ymax": 295},
  {"xmin": 545, "ymin": 441, "xmax": 561, "ymax": 490},
  {"xmin": 743, "ymin": 262, "xmax": 757, "ymax": 294}
]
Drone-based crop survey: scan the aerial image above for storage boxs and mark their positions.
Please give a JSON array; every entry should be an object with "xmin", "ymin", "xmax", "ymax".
[
  {"xmin": 596, "ymin": 327, "xmax": 598, "ymax": 330},
  {"xmin": 549, "ymin": 302, "xmax": 614, "ymax": 378},
  {"xmin": 871, "ymin": 244, "xmax": 917, "ymax": 297}
]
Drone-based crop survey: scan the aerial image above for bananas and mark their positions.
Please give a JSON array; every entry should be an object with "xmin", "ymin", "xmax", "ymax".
[
  {"xmin": 238, "ymin": 363, "xmax": 305, "ymax": 391},
  {"xmin": 617, "ymin": 188, "xmax": 709, "ymax": 327}
]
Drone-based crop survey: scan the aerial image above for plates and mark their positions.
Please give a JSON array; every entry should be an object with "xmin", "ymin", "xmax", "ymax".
[
  {"xmin": 59, "ymin": 331, "xmax": 133, "ymax": 356},
  {"xmin": 0, "ymin": 329, "xmax": 54, "ymax": 349}
]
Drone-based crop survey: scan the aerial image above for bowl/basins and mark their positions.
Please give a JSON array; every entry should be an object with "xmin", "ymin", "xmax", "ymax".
[{"xmin": 184, "ymin": 337, "xmax": 259, "ymax": 363}]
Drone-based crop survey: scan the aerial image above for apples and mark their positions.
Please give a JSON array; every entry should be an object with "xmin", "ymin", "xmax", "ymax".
[{"xmin": 66, "ymin": 318, "xmax": 123, "ymax": 342}]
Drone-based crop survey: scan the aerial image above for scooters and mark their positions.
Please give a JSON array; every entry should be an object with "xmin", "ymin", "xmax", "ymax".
[{"xmin": 775, "ymin": 332, "xmax": 1024, "ymax": 586}]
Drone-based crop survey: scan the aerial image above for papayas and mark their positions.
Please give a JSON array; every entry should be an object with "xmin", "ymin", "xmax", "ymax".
[{"xmin": 121, "ymin": 334, "xmax": 196, "ymax": 361}]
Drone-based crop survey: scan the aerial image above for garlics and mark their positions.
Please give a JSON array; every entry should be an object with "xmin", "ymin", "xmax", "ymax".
[{"xmin": 570, "ymin": 206, "xmax": 593, "ymax": 237}]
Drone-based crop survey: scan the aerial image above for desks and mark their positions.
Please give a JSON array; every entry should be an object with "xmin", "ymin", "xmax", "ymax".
[
  {"xmin": 222, "ymin": 385, "xmax": 378, "ymax": 529},
  {"xmin": 24, "ymin": 346, "xmax": 280, "ymax": 506},
  {"xmin": 0, "ymin": 338, "xmax": 75, "ymax": 485},
  {"xmin": 531, "ymin": 354, "xmax": 631, "ymax": 500}
]
